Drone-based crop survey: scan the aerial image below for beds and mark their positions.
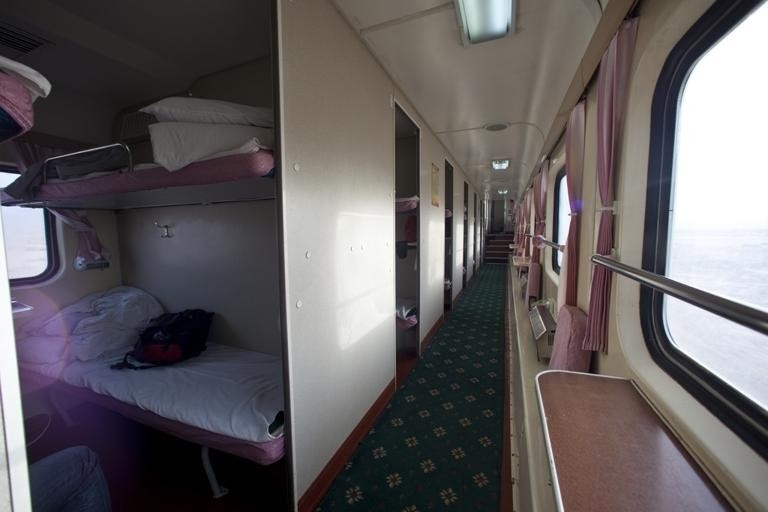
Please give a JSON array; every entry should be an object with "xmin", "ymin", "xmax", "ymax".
[
  {"xmin": 0, "ymin": 141, "xmax": 277, "ymax": 210},
  {"xmin": 18, "ymin": 288, "xmax": 289, "ymax": 500},
  {"xmin": 397, "ymin": 296, "xmax": 418, "ymax": 329},
  {"xmin": 394, "ymin": 195, "xmax": 420, "ymax": 215}
]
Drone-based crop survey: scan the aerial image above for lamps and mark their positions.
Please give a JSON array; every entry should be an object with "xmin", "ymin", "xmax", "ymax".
[
  {"xmin": 452, "ymin": 0, "xmax": 518, "ymax": 47},
  {"xmin": 493, "ymin": 160, "xmax": 510, "ymax": 170}
]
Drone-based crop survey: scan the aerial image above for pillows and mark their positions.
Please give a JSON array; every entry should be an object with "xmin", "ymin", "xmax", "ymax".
[
  {"xmin": 18, "ymin": 335, "xmax": 66, "ymax": 365},
  {"xmin": 141, "ymin": 96, "xmax": 274, "ymax": 127}
]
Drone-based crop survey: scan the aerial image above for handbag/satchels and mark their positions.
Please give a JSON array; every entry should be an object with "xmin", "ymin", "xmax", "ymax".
[{"xmin": 133, "ymin": 308, "xmax": 215, "ymax": 365}]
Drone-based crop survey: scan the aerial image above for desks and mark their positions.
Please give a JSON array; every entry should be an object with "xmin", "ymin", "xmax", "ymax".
[
  {"xmin": 512, "ymin": 254, "xmax": 534, "ymax": 268},
  {"xmin": 535, "ymin": 370, "xmax": 745, "ymax": 512}
]
